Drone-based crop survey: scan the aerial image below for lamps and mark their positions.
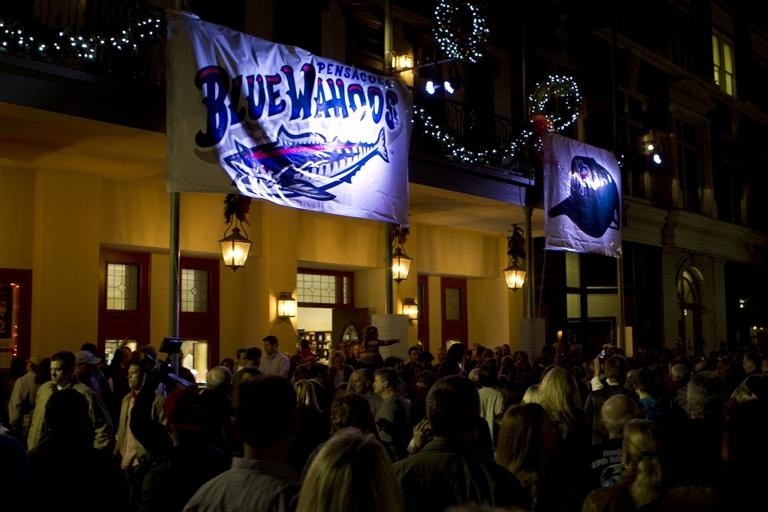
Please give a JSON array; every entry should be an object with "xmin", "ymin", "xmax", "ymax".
[
  {"xmin": 389, "ymin": 221, "xmax": 415, "ymax": 286},
  {"xmin": 402, "ymin": 297, "xmax": 419, "ymax": 322},
  {"xmin": 216, "ymin": 194, "xmax": 254, "ymax": 272},
  {"xmin": 277, "ymin": 289, "xmax": 296, "ymax": 318},
  {"xmin": 502, "ymin": 222, "xmax": 529, "ymax": 292}
]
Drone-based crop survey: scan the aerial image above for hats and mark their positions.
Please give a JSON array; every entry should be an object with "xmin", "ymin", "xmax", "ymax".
[{"xmin": 75, "ymin": 351, "xmax": 102, "ymax": 364}]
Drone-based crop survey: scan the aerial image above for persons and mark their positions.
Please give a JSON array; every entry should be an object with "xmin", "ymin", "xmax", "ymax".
[{"xmin": 0, "ymin": 325, "xmax": 768, "ymax": 512}]
